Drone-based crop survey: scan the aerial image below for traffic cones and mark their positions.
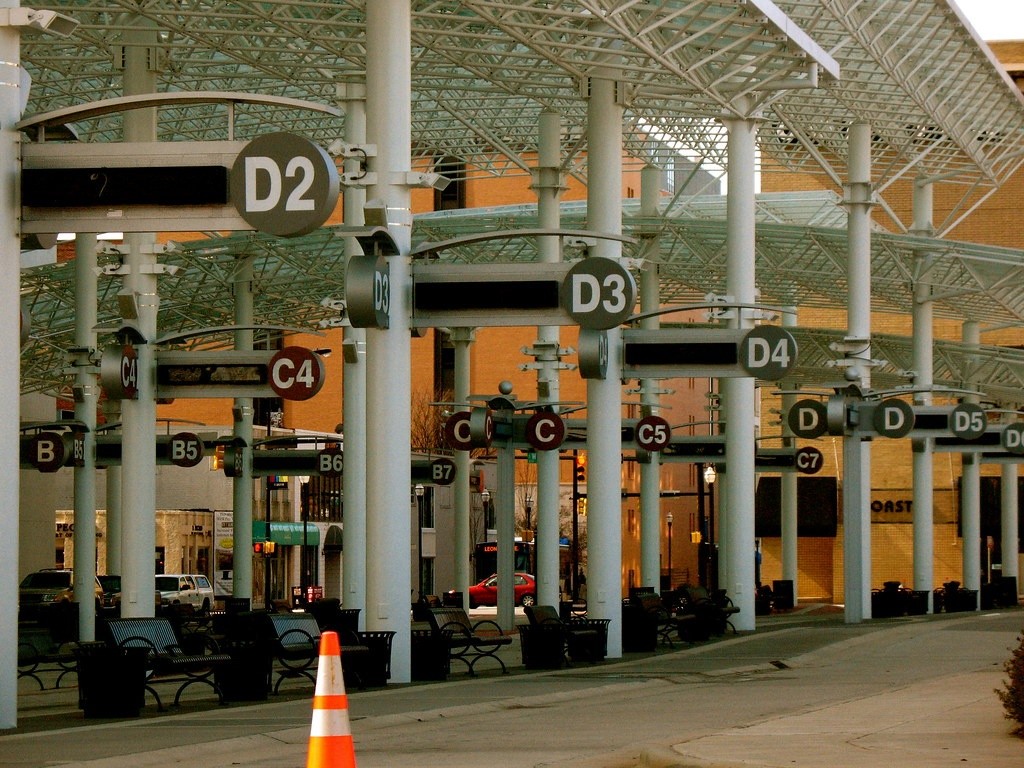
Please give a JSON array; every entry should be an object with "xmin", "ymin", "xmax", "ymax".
[{"xmin": 304, "ymin": 630, "xmax": 356, "ymax": 768}]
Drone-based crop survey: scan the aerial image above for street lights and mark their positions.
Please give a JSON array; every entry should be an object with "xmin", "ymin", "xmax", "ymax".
[
  {"xmin": 666, "ymin": 510, "xmax": 675, "ymax": 591},
  {"xmin": 524, "ymin": 493, "xmax": 533, "ymax": 574},
  {"xmin": 299, "ymin": 475, "xmax": 310, "ymax": 611},
  {"xmin": 704, "ymin": 465, "xmax": 716, "ymax": 594},
  {"xmin": 481, "ymin": 485, "xmax": 490, "ymax": 542},
  {"xmin": 414, "ymin": 483, "xmax": 425, "ymax": 602}
]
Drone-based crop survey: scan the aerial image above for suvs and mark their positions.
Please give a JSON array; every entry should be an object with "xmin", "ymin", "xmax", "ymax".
[{"xmin": 19, "ymin": 565, "xmax": 106, "ymax": 627}]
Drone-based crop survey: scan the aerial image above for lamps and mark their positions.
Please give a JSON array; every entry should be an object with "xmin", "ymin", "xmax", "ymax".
[
  {"xmin": 73, "ymin": 381, "xmax": 84, "ymax": 405},
  {"xmin": 409, "ymin": 172, "xmax": 450, "ymax": 191},
  {"xmin": 342, "ymin": 337, "xmax": 360, "ymax": 366},
  {"xmin": 9, "ymin": 7, "xmax": 81, "ymax": 40},
  {"xmin": 230, "ymin": 403, "xmax": 243, "ymax": 422},
  {"xmin": 623, "ymin": 257, "xmax": 655, "ymax": 272},
  {"xmin": 752, "ymin": 310, "xmax": 780, "ymax": 321},
  {"xmin": 118, "ymin": 287, "xmax": 140, "ymax": 321}
]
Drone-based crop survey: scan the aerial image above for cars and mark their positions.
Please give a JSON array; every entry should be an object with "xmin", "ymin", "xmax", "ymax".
[
  {"xmin": 96, "ymin": 575, "xmax": 121, "ymax": 619},
  {"xmin": 469, "ymin": 573, "xmax": 537, "ymax": 610}
]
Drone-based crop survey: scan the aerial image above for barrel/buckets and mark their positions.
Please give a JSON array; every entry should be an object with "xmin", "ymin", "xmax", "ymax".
[
  {"xmin": 773, "ymin": 580, "xmax": 794, "ymax": 610},
  {"xmin": 443, "ymin": 592, "xmax": 464, "ymax": 608}
]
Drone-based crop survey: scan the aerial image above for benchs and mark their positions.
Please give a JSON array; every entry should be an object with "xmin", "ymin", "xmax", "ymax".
[
  {"xmin": 427, "ymin": 607, "xmax": 514, "ymax": 677},
  {"xmin": 516, "ymin": 604, "xmax": 610, "ymax": 669},
  {"xmin": 422, "ymin": 593, "xmax": 451, "ymax": 608},
  {"xmin": 872, "ymin": 580, "xmax": 977, "ymax": 617},
  {"xmin": 17, "ymin": 599, "xmax": 369, "ymax": 713},
  {"xmin": 623, "ymin": 582, "xmax": 741, "ymax": 651}
]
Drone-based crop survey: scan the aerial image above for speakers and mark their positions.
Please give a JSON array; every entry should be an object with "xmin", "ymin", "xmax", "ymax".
[
  {"xmin": 642, "ymin": 404, "xmax": 652, "ymax": 418},
  {"xmin": 538, "ymin": 377, "xmax": 550, "ymax": 397},
  {"xmin": 342, "ymin": 338, "xmax": 359, "ymax": 363},
  {"xmin": 72, "ymin": 383, "xmax": 86, "ymax": 403}
]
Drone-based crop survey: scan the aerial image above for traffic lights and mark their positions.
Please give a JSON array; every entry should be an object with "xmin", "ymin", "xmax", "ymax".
[{"xmin": 254, "ymin": 541, "xmax": 263, "ymax": 553}]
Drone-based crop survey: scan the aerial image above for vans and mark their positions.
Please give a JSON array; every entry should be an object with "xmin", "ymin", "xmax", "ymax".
[{"xmin": 155, "ymin": 574, "xmax": 215, "ymax": 618}]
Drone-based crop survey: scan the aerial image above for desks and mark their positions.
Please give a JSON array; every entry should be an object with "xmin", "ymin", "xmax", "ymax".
[
  {"xmin": 517, "ymin": 624, "xmax": 571, "ymax": 670},
  {"xmin": 570, "ymin": 619, "xmax": 612, "ymax": 664},
  {"xmin": 69, "ymin": 644, "xmax": 153, "ymax": 717},
  {"xmin": 408, "ymin": 629, "xmax": 455, "ymax": 681},
  {"xmin": 342, "ymin": 630, "xmax": 395, "ymax": 690},
  {"xmin": 208, "ymin": 634, "xmax": 280, "ymax": 702}
]
